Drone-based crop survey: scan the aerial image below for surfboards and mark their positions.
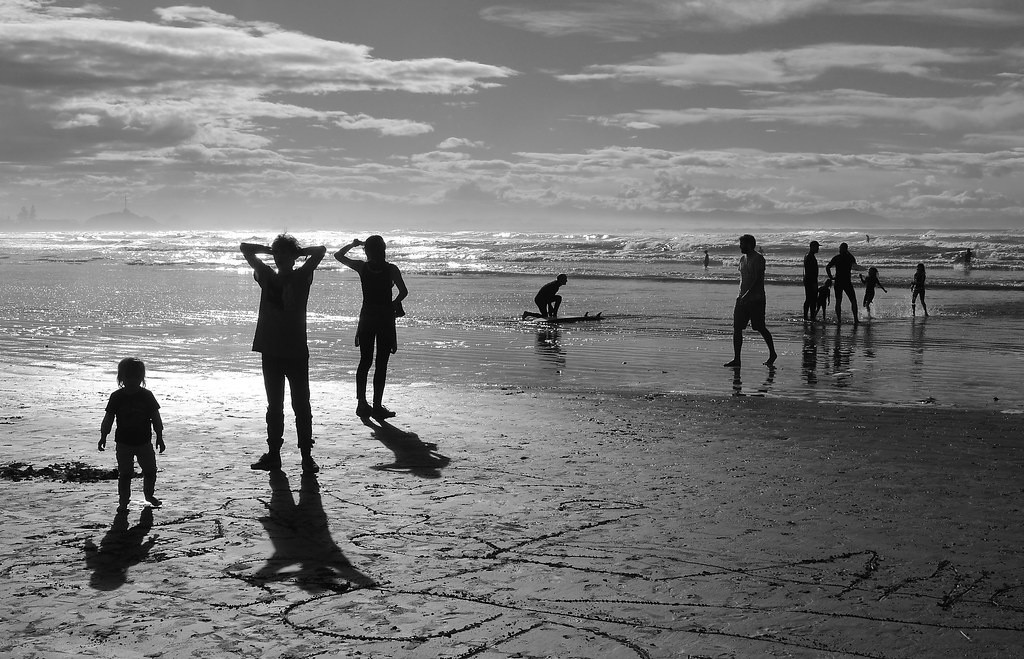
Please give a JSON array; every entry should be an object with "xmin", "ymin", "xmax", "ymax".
[{"xmin": 532, "ymin": 311, "xmax": 607, "ymax": 323}]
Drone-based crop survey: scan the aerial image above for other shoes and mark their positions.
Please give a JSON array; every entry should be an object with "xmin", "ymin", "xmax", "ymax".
[
  {"xmin": 356, "ymin": 404, "xmax": 373, "ymax": 418},
  {"xmin": 250, "ymin": 453, "xmax": 282, "ymax": 471},
  {"xmin": 301, "ymin": 456, "xmax": 319, "ymax": 473},
  {"xmin": 373, "ymin": 405, "xmax": 396, "ymax": 420}
]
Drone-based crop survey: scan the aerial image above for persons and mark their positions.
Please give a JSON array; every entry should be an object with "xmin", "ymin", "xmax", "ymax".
[
  {"xmin": 866, "ymin": 235, "xmax": 869, "ymax": 242},
  {"xmin": 911, "ymin": 262, "xmax": 930, "ymax": 317},
  {"xmin": 826, "ymin": 244, "xmax": 862, "ymax": 324},
  {"xmin": 803, "ymin": 241, "xmax": 823, "ymax": 321},
  {"xmin": 240, "ymin": 234, "xmax": 326, "ymax": 473},
  {"xmin": 723, "ymin": 235, "xmax": 777, "ymax": 367},
  {"xmin": 334, "ymin": 236, "xmax": 408, "ymax": 418},
  {"xmin": 98, "ymin": 358, "xmax": 166, "ymax": 513},
  {"xmin": 522, "ymin": 274, "xmax": 567, "ymax": 320},
  {"xmin": 964, "ymin": 248, "xmax": 973, "ymax": 262},
  {"xmin": 814, "ymin": 279, "xmax": 833, "ymax": 320},
  {"xmin": 860, "ymin": 267, "xmax": 888, "ymax": 317},
  {"xmin": 704, "ymin": 250, "xmax": 709, "ymax": 270}
]
